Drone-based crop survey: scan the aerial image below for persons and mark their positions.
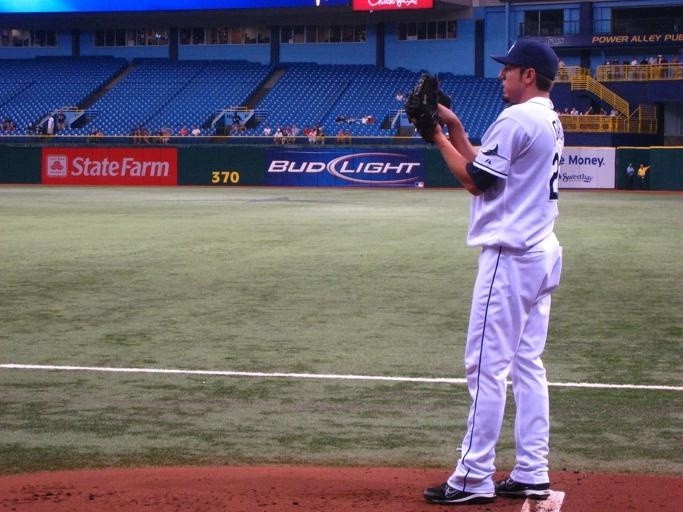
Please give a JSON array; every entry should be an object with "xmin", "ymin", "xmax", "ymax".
[
  {"xmin": 366, "ymin": 116, "xmax": 375, "ymax": 125},
  {"xmin": 192, "ymin": 124, "xmax": 202, "ymax": 137},
  {"xmin": 556, "ymin": 104, "xmax": 622, "ymax": 130},
  {"xmin": 336, "ymin": 114, "xmax": 349, "ymax": 124},
  {"xmin": 626, "ymin": 161, "xmax": 635, "ymax": 190},
  {"xmin": 174, "ymin": 127, "xmax": 189, "ymax": 136},
  {"xmin": 96, "ymin": 128, "xmax": 106, "ymax": 136},
  {"xmin": 232, "ymin": 112, "xmax": 242, "ymax": 130},
  {"xmin": 273, "ymin": 126, "xmax": 284, "ymax": 144},
  {"xmin": 292, "ymin": 125, "xmax": 300, "ymax": 133},
  {"xmin": 337, "ymin": 128, "xmax": 344, "ymax": 146},
  {"xmin": 403, "ymin": 32, "xmax": 579, "ymax": 507},
  {"xmin": 637, "ymin": 163, "xmax": 651, "ymax": 190},
  {"xmin": 1, "ymin": 118, "xmax": 17, "ymax": 131},
  {"xmin": 263, "ymin": 124, "xmax": 273, "ymax": 135},
  {"xmin": 285, "ymin": 125, "xmax": 291, "ymax": 133},
  {"xmin": 47, "ymin": 113, "xmax": 55, "ymax": 135},
  {"xmin": 287, "ymin": 128, "xmax": 296, "ymax": 138},
  {"xmin": 558, "ymin": 57, "xmax": 566, "ymax": 68},
  {"xmin": 602, "ymin": 52, "xmax": 671, "ymax": 79},
  {"xmin": 344, "ymin": 128, "xmax": 352, "ymax": 145},
  {"xmin": 238, "ymin": 123, "xmax": 246, "ymax": 131},
  {"xmin": 127, "ymin": 125, "xmax": 172, "ymax": 145},
  {"xmin": 303, "ymin": 122, "xmax": 327, "ymax": 145},
  {"xmin": 56, "ymin": 109, "xmax": 66, "ymax": 130}
]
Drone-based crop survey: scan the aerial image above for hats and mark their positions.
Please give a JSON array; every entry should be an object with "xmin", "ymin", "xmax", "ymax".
[{"xmin": 488, "ymin": 37, "xmax": 559, "ymax": 81}]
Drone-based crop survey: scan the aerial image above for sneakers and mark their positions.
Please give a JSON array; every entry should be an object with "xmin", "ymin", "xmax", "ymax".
[
  {"xmin": 494, "ymin": 475, "xmax": 551, "ymax": 500},
  {"xmin": 422, "ymin": 477, "xmax": 497, "ymax": 505}
]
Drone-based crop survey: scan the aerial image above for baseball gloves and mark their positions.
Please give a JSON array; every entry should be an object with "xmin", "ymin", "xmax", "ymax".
[{"xmin": 404, "ymin": 74, "xmax": 452, "ymax": 145}]
